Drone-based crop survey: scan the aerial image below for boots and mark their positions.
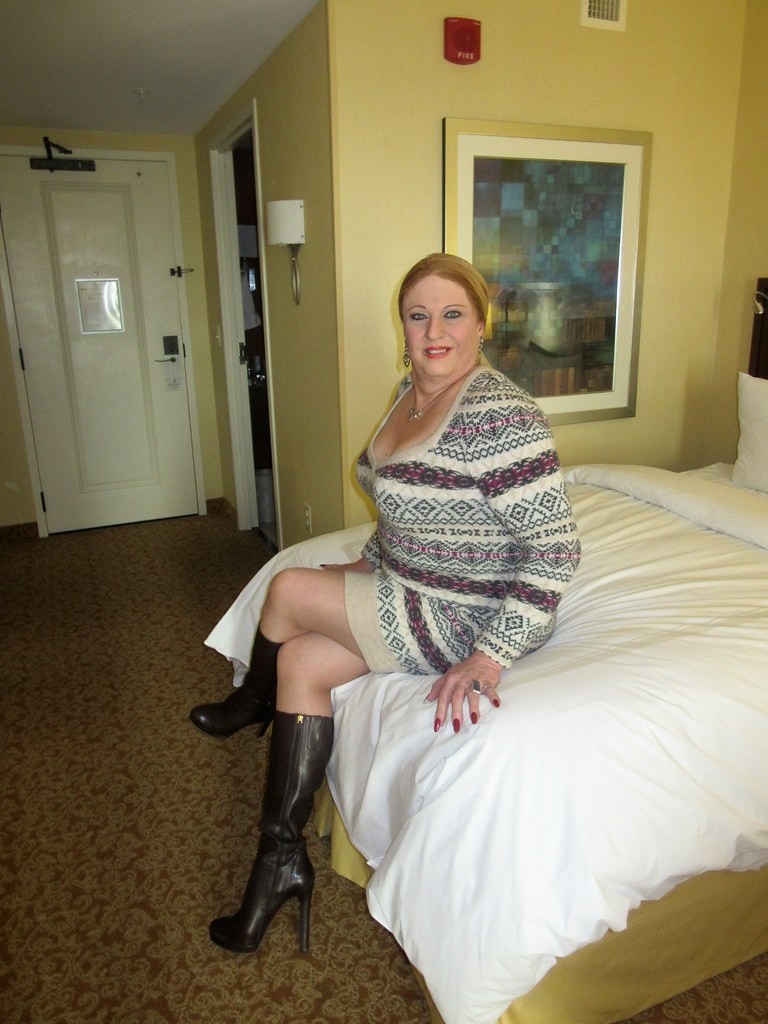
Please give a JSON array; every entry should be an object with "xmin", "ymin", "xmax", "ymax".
[
  {"xmin": 189, "ymin": 622, "xmax": 285, "ymax": 741},
  {"xmin": 209, "ymin": 711, "xmax": 334, "ymax": 954}
]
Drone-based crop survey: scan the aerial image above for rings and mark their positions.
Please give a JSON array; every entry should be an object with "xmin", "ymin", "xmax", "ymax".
[
  {"xmin": 471, "ymin": 679, "xmax": 481, "ymax": 694},
  {"xmin": 482, "ymin": 683, "xmax": 492, "ymax": 692}
]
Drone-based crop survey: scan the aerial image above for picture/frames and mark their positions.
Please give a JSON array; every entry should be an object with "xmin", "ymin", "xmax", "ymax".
[{"xmin": 441, "ymin": 118, "xmax": 655, "ymax": 422}]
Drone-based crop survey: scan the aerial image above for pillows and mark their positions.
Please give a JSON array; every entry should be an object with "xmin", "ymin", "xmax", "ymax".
[{"xmin": 731, "ymin": 371, "xmax": 768, "ymax": 494}]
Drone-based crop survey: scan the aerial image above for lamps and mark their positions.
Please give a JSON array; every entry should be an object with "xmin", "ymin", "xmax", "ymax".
[
  {"xmin": 750, "ymin": 292, "xmax": 768, "ymax": 314},
  {"xmin": 264, "ymin": 199, "xmax": 306, "ymax": 305}
]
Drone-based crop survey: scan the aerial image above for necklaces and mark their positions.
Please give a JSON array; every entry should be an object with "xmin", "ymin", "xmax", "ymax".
[{"xmin": 408, "ymin": 391, "xmax": 443, "ymax": 422}]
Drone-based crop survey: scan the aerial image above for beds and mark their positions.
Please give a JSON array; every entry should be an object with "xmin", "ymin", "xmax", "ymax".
[{"xmin": 203, "ymin": 277, "xmax": 768, "ymax": 1024}]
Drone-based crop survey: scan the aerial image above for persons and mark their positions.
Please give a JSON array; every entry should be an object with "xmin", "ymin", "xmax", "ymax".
[{"xmin": 190, "ymin": 252, "xmax": 582, "ymax": 953}]
